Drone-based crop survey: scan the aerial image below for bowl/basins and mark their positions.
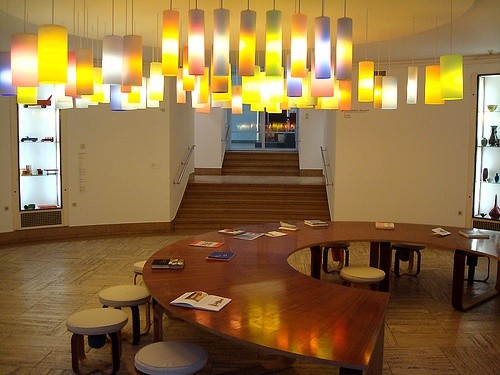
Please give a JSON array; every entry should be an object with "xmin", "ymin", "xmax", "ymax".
[{"xmin": 488, "ymin": 105, "xmax": 498, "ymax": 112}]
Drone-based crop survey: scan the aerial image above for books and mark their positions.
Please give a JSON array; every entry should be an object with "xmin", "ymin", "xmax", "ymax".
[
  {"xmin": 170, "ymin": 291, "xmax": 231, "ymax": 312},
  {"xmin": 151, "ymin": 258, "xmax": 185, "ymax": 269},
  {"xmin": 279, "ymin": 221, "xmax": 301, "ymax": 231},
  {"xmin": 304, "ymin": 220, "xmax": 329, "ymax": 227},
  {"xmin": 218, "ymin": 228, "xmax": 244, "ymax": 235},
  {"xmin": 376, "ymin": 221, "xmax": 394, "ymax": 229},
  {"xmin": 458, "ymin": 229, "xmax": 490, "ymax": 238},
  {"xmin": 189, "ymin": 240, "xmax": 224, "ymax": 248},
  {"xmin": 206, "ymin": 251, "xmax": 236, "ymax": 262},
  {"xmin": 432, "ymin": 227, "xmax": 451, "ymax": 237},
  {"xmin": 233, "ymin": 231, "xmax": 265, "ymax": 241}
]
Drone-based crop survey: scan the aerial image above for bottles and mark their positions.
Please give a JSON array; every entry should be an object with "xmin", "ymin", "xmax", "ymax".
[
  {"xmin": 489, "ymin": 194, "xmax": 500, "ymax": 219},
  {"xmin": 495, "ymin": 173, "xmax": 500, "ymax": 183},
  {"xmin": 481, "ymin": 138, "xmax": 487, "ymax": 147},
  {"xmin": 482, "ymin": 168, "xmax": 488, "ymax": 181},
  {"xmin": 489, "ymin": 125, "xmax": 500, "ymax": 147}
]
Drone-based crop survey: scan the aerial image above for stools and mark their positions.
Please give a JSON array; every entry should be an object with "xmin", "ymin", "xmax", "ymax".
[
  {"xmin": 320, "ymin": 242, "xmax": 350, "ymax": 275},
  {"xmin": 340, "ymin": 267, "xmax": 385, "ymax": 290},
  {"xmin": 389, "ymin": 242, "xmax": 425, "ymax": 277},
  {"xmin": 464, "ymin": 252, "xmax": 493, "ymax": 282},
  {"xmin": 66, "ymin": 258, "xmax": 210, "ymax": 375}
]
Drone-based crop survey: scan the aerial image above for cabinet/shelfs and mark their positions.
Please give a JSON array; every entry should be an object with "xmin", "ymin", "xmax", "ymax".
[
  {"xmin": 19, "ymin": 136, "xmax": 60, "ymax": 209},
  {"xmin": 473, "ymin": 109, "xmax": 500, "ymax": 221}
]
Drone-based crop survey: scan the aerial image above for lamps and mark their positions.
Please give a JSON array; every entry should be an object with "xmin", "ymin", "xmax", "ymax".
[
  {"xmin": 424, "ymin": 0, "xmax": 463, "ymax": 105},
  {"xmin": 0, "ymin": 0, "xmax": 397, "ymax": 114},
  {"xmin": 406, "ymin": 0, "xmax": 418, "ymax": 104}
]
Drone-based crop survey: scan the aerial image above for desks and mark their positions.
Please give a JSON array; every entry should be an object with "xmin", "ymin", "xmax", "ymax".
[{"xmin": 142, "ymin": 221, "xmax": 500, "ymax": 374}]
